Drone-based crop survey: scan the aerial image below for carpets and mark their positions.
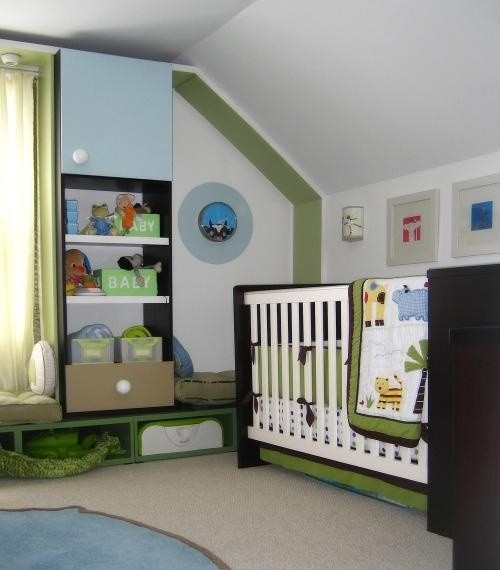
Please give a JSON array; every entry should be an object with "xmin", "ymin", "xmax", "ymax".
[{"xmin": 0, "ymin": 496, "xmax": 231, "ymax": 570}]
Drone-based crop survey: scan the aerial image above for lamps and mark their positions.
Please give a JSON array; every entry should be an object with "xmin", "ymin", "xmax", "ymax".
[{"xmin": 341, "ymin": 204, "xmax": 365, "ymax": 242}]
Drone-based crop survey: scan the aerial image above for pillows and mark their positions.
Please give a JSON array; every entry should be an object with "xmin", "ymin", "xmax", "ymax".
[
  {"xmin": 167, "ymin": 330, "xmax": 194, "ymax": 383},
  {"xmin": 24, "ymin": 339, "xmax": 58, "ymax": 399}
]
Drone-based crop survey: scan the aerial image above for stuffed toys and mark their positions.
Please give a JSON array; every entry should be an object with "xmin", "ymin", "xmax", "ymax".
[
  {"xmin": 65, "ymin": 249, "xmax": 96, "ymax": 295},
  {"xmin": 108, "ymin": 194, "xmax": 135, "ymax": 236},
  {"xmin": 80, "ymin": 204, "xmax": 118, "ymax": 236},
  {"xmin": 118, "ymin": 254, "xmax": 162, "ymax": 287}
]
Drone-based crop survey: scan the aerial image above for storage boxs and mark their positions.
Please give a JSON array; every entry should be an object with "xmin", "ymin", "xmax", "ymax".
[
  {"xmin": 65, "ymin": 335, "xmax": 118, "ymax": 366},
  {"xmin": 90, "ymin": 266, "xmax": 159, "ymax": 297},
  {"xmin": 111, "ymin": 209, "xmax": 162, "ymax": 239},
  {"xmin": 114, "ymin": 336, "xmax": 164, "ymax": 364}
]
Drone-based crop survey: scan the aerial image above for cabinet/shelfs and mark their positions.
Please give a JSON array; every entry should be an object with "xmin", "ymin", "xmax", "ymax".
[
  {"xmin": 40, "ymin": 44, "xmax": 180, "ymax": 413},
  {"xmin": 0, "ymin": 396, "xmax": 241, "ymax": 481}
]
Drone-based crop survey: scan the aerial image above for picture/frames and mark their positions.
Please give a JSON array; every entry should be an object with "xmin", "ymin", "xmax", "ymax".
[
  {"xmin": 447, "ymin": 170, "xmax": 500, "ymax": 261},
  {"xmin": 384, "ymin": 186, "xmax": 442, "ymax": 267}
]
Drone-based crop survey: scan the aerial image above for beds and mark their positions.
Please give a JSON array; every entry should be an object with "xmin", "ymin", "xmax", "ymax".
[{"xmin": 226, "ymin": 261, "xmax": 499, "ymax": 569}]
